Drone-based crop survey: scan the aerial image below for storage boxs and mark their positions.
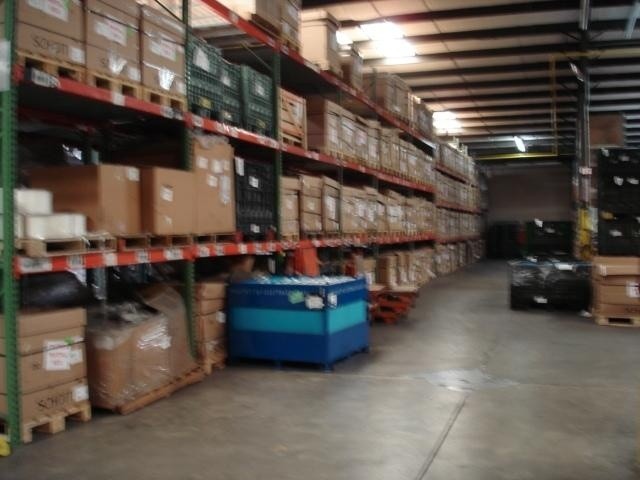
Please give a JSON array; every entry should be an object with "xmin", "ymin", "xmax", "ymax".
[{"xmin": 590, "ymin": 256, "xmax": 640, "ymax": 317}]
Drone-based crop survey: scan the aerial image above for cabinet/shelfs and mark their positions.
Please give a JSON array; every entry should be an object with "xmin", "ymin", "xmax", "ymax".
[{"xmin": 1, "ymin": 0, "xmax": 478, "ymax": 456}]
